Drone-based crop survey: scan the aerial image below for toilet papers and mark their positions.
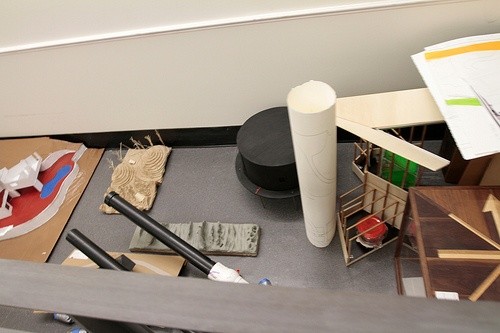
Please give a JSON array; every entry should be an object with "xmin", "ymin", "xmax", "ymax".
[{"xmin": 286, "ymin": 80, "xmax": 337, "ymax": 248}]
